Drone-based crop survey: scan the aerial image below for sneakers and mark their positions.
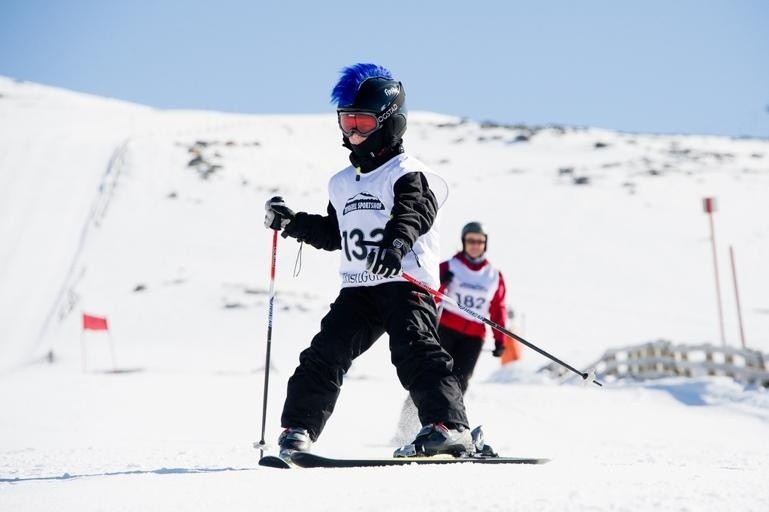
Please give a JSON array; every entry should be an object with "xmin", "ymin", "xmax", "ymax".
[
  {"xmin": 412, "ymin": 422, "xmax": 473, "ymax": 456},
  {"xmin": 278, "ymin": 427, "xmax": 311, "ymax": 452}
]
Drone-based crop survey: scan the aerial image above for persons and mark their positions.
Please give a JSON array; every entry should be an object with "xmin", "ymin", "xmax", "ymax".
[
  {"xmin": 435, "ymin": 220, "xmax": 509, "ymax": 397},
  {"xmin": 261, "ymin": 62, "xmax": 477, "ymax": 463}
]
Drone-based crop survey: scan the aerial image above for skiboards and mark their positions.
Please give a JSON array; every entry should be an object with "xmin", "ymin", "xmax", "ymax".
[{"xmin": 257, "ymin": 424, "xmax": 548, "ymax": 468}]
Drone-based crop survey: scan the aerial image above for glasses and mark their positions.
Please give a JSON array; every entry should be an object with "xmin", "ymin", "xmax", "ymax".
[
  {"xmin": 466, "ymin": 239, "xmax": 484, "ymax": 244},
  {"xmin": 337, "ymin": 82, "xmax": 405, "ymax": 137}
]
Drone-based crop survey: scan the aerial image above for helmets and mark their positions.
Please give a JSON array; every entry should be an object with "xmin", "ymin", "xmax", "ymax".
[
  {"xmin": 461, "ymin": 223, "xmax": 487, "ymax": 251},
  {"xmin": 333, "ymin": 63, "xmax": 407, "ymax": 156}
]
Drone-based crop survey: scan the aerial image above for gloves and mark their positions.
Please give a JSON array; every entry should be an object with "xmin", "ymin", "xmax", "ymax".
[
  {"xmin": 265, "ymin": 200, "xmax": 296, "ymax": 237},
  {"xmin": 366, "ymin": 234, "xmax": 411, "ymax": 279},
  {"xmin": 493, "ymin": 342, "xmax": 504, "ymax": 356},
  {"xmin": 441, "ymin": 271, "xmax": 454, "ymax": 285}
]
